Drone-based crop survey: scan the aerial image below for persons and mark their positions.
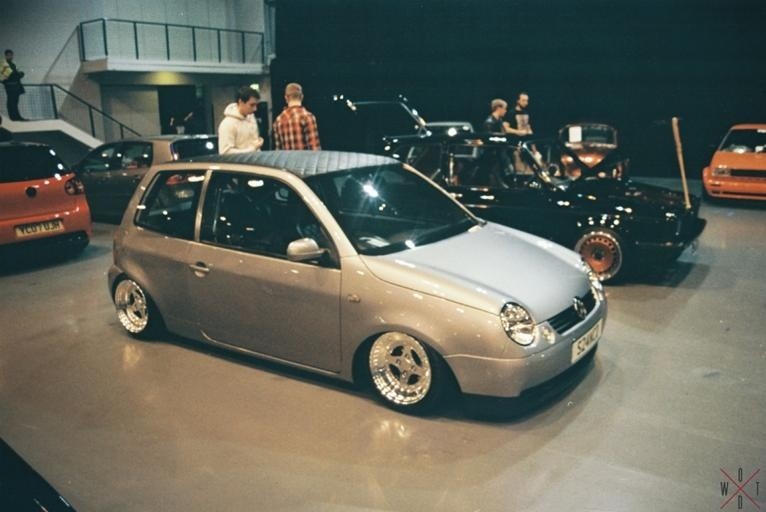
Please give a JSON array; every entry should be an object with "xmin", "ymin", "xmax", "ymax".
[
  {"xmin": 273, "ymin": 83, "xmax": 322, "ymax": 150},
  {"xmin": 217, "ymin": 87, "xmax": 264, "ymax": 155},
  {"xmin": 0, "ymin": 48, "xmax": 31, "ymax": 120},
  {"xmin": 481, "ymin": 99, "xmax": 507, "ymax": 133},
  {"xmin": 502, "ymin": 93, "xmax": 535, "ymax": 153}
]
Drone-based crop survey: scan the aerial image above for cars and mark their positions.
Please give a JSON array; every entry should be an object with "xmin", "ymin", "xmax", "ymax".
[
  {"xmin": 71, "ymin": 132, "xmax": 218, "ymax": 222},
  {"xmin": 0, "ymin": 139, "xmax": 92, "ymax": 253},
  {"xmin": 329, "ymin": 91, "xmax": 429, "ymax": 155},
  {"xmin": 370, "ymin": 128, "xmax": 710, "ymax": 283},
  {"xmin": 403, "ymin": 118, "xmax": 480, "ymax": 168},
  {"xmin": 554, "ymin": 120, "xmax": 631, "ymax": 182},
  {"xmin": 700, "ymin": 122, "xmax": 765, "ymax": 205},
  {"xmin": 106, "ymin": 151, "xmax": 610, "ymax": 415}
]
